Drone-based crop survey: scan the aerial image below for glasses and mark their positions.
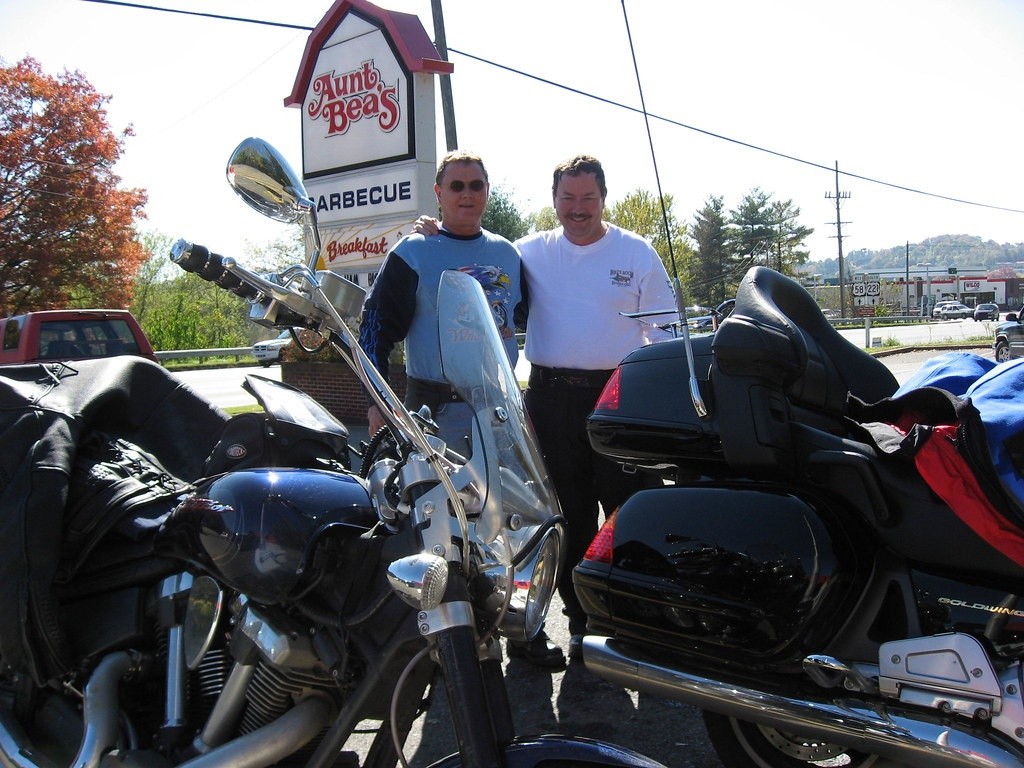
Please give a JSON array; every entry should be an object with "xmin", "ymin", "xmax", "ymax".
[{"xmin": 438, "ymin": 179, "xmax": 487, "ymax": 193}]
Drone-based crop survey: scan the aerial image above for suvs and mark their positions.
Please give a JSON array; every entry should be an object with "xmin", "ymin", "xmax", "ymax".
[
  {"xmin": 942, "ymin": 304, "xmax": 974, "ymax": 319},
  {"xmin": 248, "ymin": 324, "xmax": 311, "ymax": 369},
  {"xmin": 993, "ymin": 305, "xmax": 1024, "ymax": 361}
]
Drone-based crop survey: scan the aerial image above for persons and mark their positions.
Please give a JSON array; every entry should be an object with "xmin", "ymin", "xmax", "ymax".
[
  {"xmin": 358, "ymin": 149, "xmax": 534, "ymax": 521},
  {"xmin": 416, "ymin": 156, "xmax": 677, "ymax": 666}
]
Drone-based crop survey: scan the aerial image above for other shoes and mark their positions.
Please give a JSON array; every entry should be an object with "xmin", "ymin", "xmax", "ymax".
[{"xmin": 569, "ymin": 634, "xmax": 584, "ymax": 655}]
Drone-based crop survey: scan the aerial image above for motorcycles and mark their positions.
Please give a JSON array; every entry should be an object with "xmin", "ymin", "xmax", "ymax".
[
  {"xmin": 0, "ymin": 134, "xmax": 671, "ymax": 766},
  {"xmin": 572, "ymin": 262, "xmax": 1024, "ymax": 768}
]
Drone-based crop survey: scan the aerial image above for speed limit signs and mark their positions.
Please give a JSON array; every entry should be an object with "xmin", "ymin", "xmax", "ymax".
[
  {"xmin": 866, "ymin": 274, "xmax": 880, "ymax": 296},
  {"xmin": 852, "ymin": 273, "xmax": 866, "ymax": 297}
]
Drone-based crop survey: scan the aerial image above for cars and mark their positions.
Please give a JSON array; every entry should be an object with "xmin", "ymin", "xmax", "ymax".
[
  {"xmin": 932, "ymin": 300, "xmax": 960, "ymax": 318},
  {"xmin": 0, "ymin": 308, "xmax": 159, "ymax": 378},
  {"xmin": 975, "ymin": 303, "xmax": 1000, "ymax": 321}
]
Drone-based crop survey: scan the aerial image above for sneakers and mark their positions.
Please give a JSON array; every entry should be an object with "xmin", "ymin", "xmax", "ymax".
[{"xmin": 506, "ymin": 629, "xmax": 566, "ymax": 667}]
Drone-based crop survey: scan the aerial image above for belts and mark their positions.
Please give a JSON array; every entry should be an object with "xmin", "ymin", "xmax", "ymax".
[{"xmin": 530, "ymin": 364, "xmax": 617, "ymax": 389}]
